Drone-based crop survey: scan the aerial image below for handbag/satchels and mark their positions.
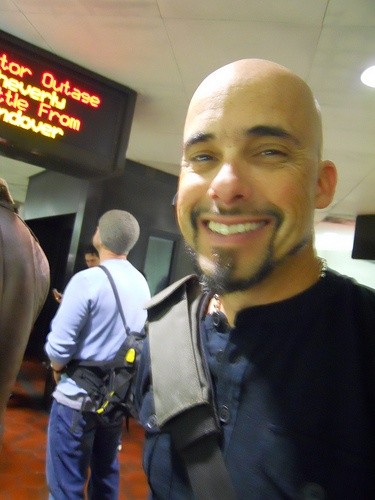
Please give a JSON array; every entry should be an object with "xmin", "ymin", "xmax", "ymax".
[{"xmin": 96, "ymin": 331, "xmax": 150, "ymax": 428}]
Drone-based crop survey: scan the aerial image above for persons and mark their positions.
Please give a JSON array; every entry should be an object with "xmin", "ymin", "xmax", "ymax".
[
  {"xmin": 42, "ymin": 211, "xmax": 151, "ymax": 500},
  {"xmin": 130, "ymin": 56, "xmax": 375, "ymax": 500},
  {"xmin": 0, "ymin": 178, "xmax": 50, "ymax": 418}
]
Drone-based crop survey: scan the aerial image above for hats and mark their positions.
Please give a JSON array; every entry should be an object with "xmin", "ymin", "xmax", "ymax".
[{"xmin": 98, "ymin": 210, "xmax": 140, "ymax": 256}]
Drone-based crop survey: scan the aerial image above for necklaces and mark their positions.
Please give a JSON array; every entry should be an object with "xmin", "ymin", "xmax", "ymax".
[{"xmin": 214, "ymin": 257, "xmax": 328, "ymax": 313}]
